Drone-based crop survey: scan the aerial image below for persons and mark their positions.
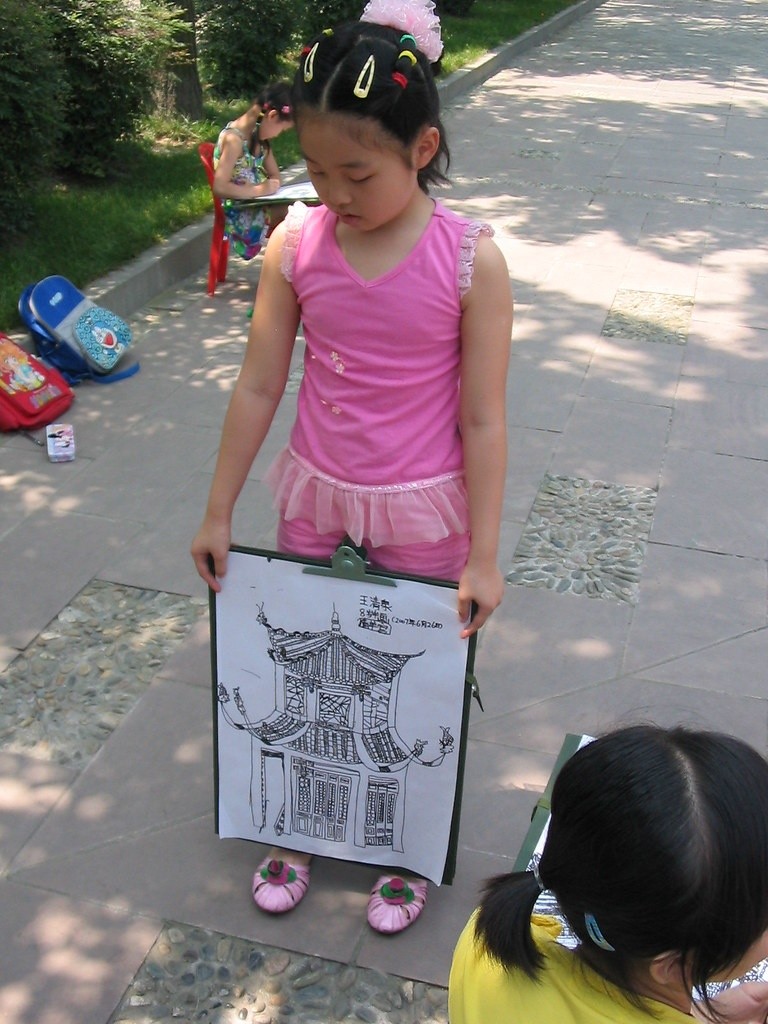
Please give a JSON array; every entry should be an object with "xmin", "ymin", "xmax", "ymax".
[
  {"xmin": 445, "ymin": 720, "xmax": 767, "ymax": 1024},
  {"xmin": 213, "ymin": 79, "xmax": 320, "ymax": 266},
  {"xmin": 186, "ymin": 6, "xmax": 514, "ymax": 937}
]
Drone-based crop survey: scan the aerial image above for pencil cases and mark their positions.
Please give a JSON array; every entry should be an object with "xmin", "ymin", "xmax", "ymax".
[{"xmin": 46, "ymin": 423, "xmax": 76, "ymax": 462}]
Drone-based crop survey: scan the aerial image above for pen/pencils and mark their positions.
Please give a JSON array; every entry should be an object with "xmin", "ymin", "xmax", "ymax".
[{"xmin": 258, "ymin": 167, "xmax": 270, "ymax": 180}]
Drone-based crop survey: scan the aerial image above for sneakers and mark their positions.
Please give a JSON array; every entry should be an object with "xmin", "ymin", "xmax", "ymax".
[
  {"xmin": 368, "ymin": 868, "xmax": 428, "ymax": 932},
  {"xmin": 250, "ymin": 845, "xmax": 311, "ymax": 911}
]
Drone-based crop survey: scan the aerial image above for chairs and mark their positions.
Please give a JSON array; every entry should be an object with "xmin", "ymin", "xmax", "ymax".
[{"xmin": 197, "ymin": 142, "xmax": 274, "ymax": 297}]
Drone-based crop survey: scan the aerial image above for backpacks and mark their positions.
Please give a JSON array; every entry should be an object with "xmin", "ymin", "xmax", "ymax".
[
  {"xmin": 18, "ymin": 275, "xmax": 134, "ymax": 384},
  {"xmin": 0, "ymin": 332, "xmax": 74, "ymax": 433}
]
